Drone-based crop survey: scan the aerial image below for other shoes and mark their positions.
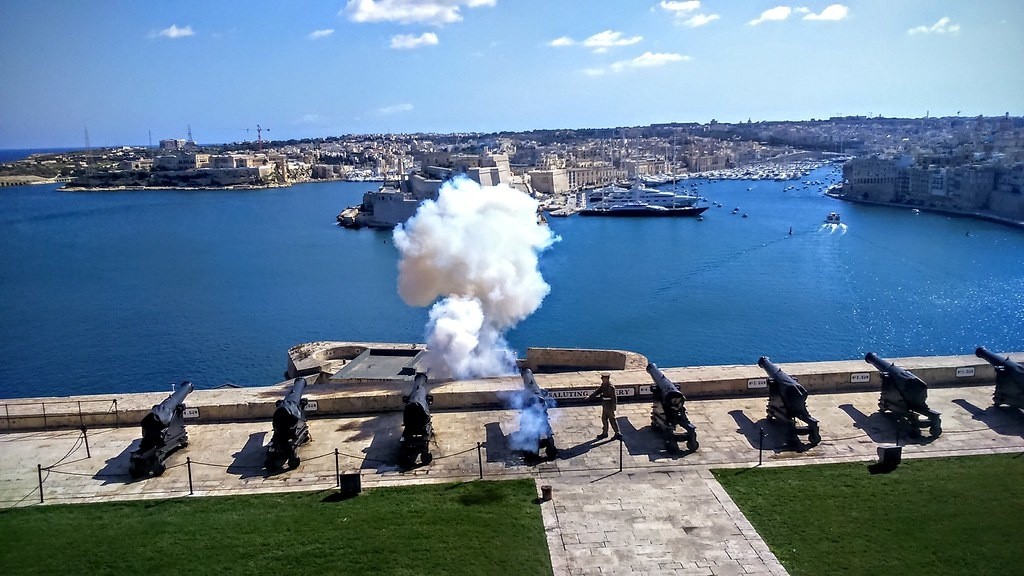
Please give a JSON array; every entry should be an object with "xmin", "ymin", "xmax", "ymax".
[
  {"xmin": 611, "ymin": 433, "xmax": 619, "ymax": 441},
  {"xmin": 597, "ymin": 433, "xmax": 608, "ymax": 438}
]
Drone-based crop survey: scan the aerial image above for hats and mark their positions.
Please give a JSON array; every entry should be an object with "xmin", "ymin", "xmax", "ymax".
[{"xmin": 600, "ymin": 372, "xmax": 611, "ymax": 378}]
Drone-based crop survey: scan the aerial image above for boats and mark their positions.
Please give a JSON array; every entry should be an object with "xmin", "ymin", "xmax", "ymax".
[{"xmin": 536, "ymin": 125, "xmax": 851, "ymax": 224}]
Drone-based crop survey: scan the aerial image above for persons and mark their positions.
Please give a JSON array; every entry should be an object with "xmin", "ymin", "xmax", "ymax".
[{"xmin": 585, "ymin": 375, "xmax": 623, "ymax": 441}]
